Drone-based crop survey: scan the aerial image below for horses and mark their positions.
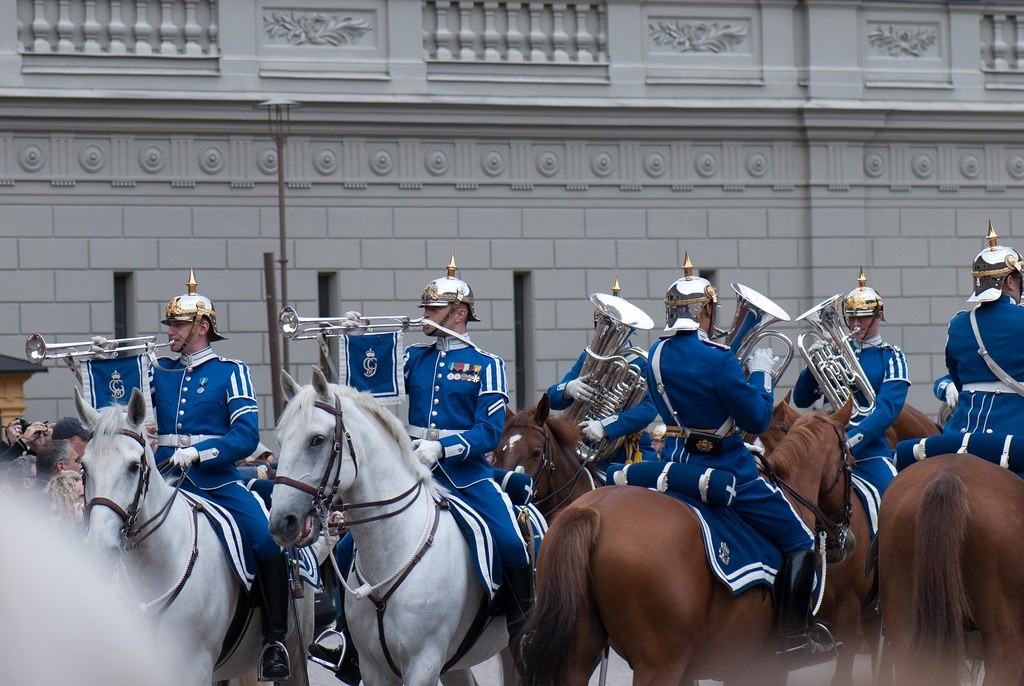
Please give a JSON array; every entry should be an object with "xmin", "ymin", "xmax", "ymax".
[
  {"xmin": 515, "ymin": 392, "xmax": 860, "ymax": 686},
  {"xmin": 877, "ymin": 451, "xmax": 1024, "ymax": 686},
  {"xmin": 742, "ymin": 386, "xmax": 948, "ymax": 686},
  {"xmin": 267, "ymin": 364, "xmax": 550, "ymax": 686},
  {"xmin": 73, "ymin": 383, "xmax": 319, "ymax": 686},
  {"xmin": 491, "ymin": 388, "xmax": 608, "ymax": 531}
]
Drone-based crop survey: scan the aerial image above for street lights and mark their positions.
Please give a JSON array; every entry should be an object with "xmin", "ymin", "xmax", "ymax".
[{"xmin": 255, "ymin": 97, "xmax": 304, "ymax": 375}]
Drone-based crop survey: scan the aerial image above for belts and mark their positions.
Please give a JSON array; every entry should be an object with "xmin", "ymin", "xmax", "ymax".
[
  {"xmin": 962, "ymin": 380, "xmax": 1024, "ymax": 394},
  {"xmin": 156, "ymin": 434, "xmax": 228, "ymax": 448},
  {"xmin": 406, "ymin": 424, "xmax": 471, "ymax": 442},
  {"xmin": 821, "ymin": 402, "xmax": 871, "ymax": 418}
]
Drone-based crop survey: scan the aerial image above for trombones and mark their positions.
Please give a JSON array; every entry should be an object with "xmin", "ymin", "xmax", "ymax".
[
  {"xmin": 23, "ymin": 331, "xmax": 177, "ymax": 365},
  {"xmin": 278, "ymin": 304, "xmax": 429, "ymax": 341}
]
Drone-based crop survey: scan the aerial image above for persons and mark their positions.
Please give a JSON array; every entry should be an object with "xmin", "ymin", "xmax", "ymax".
[
  {"xmin": 307, "ymin": 247, "xmax": 534, "ymax": 686},
  {"xmin": 544, "ymin": 220, "xmax": 1024, "ymax": 631},
  {"xmin": 0, "ymin": 269, "xmax": 346, "ymax": 677}
]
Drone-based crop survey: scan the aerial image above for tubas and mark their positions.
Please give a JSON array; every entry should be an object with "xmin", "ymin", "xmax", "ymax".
[
  {"xmin": 793, "ymin": 292, "xmax": 878, "ymax": 429},
  {"xmin": 721, "ymin": 279, "xmax": 796, "ymax": 387},
  {"xmin": 561, "ymin": 290, "xmax": 655, "ymax": 465}
]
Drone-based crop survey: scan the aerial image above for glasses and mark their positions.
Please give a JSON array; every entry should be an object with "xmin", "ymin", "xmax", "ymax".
[{"xmin": 55, "ymin": 456, "xmax": 82, "ymax": 467}]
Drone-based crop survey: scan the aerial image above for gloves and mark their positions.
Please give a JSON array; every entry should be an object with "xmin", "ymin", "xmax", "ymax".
[
  {"xmin": 945, "ymin": 382, "xmax": 959, "ymax": 408},
  {"xmin": 808, "ymin": 339, "xmax": 829, "ymax": 365},
  {"xmin": 88, "ymin": 336, "xmax": 119, "ymax": 360},
  {"xmin": 169, "ymin": 447, "xmax": 199, "ymax": 469},
  {"xmin": 410, "ymin": 439, "xmax": 443, "ymax": 468},
  {"xmin": 577, "ymin": 420, "xmax": 605, "ymax": 443},
  {"xmin": 745, "ymin": 347, "xmax": 779, "ymax": 374},
  {"xmin": 341, "ymin": 310, "xmax": 371, "ymax": 336},
  {"xmin": 565, "ymin": 375, "xmax": 596, "ymax": 403}
]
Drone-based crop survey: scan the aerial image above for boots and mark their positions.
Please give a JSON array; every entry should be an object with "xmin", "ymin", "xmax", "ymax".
[
  {"xmin": 773, "ymin": 550, "xmax": 847, "ymax": 671},
  {"xmin": 307, "ymin": 622, "xmax": 362, "ymax": 686},
  {"xmin": 501, "ymin": 561, "xmax": 537, "ymax": 680},
  {"xmin": 251, "ymin": 546, "xmax": 293, "ymax": 677}
]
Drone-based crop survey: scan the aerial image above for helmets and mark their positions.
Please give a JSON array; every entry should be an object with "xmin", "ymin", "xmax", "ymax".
[
  {"xmin": 664, "ymin": 248, "xmax": 723, "ymax": 324},
  {"xmin": 841, "ymin": 265, "xmax": 887, "ymax": 323},
  {"xmin": 417, "ymin": 246, "xmax": 481, "ymax": 321},
  {"xmin": 964, "ymin": 217, "xmax": 1024, "ymax": 303},
  {"xmin": 161, "ymin": 267, "xmax": 229, "ymax": 341}
]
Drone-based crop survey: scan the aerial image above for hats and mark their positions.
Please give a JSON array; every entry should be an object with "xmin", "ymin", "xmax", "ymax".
[
  {"xmin": 593, "ymin": 274, "xmax": 622, "ymax": 329},
  {"xmin": 52, "ymin": 416, "xmax": 89, "ymax": 440}
]
